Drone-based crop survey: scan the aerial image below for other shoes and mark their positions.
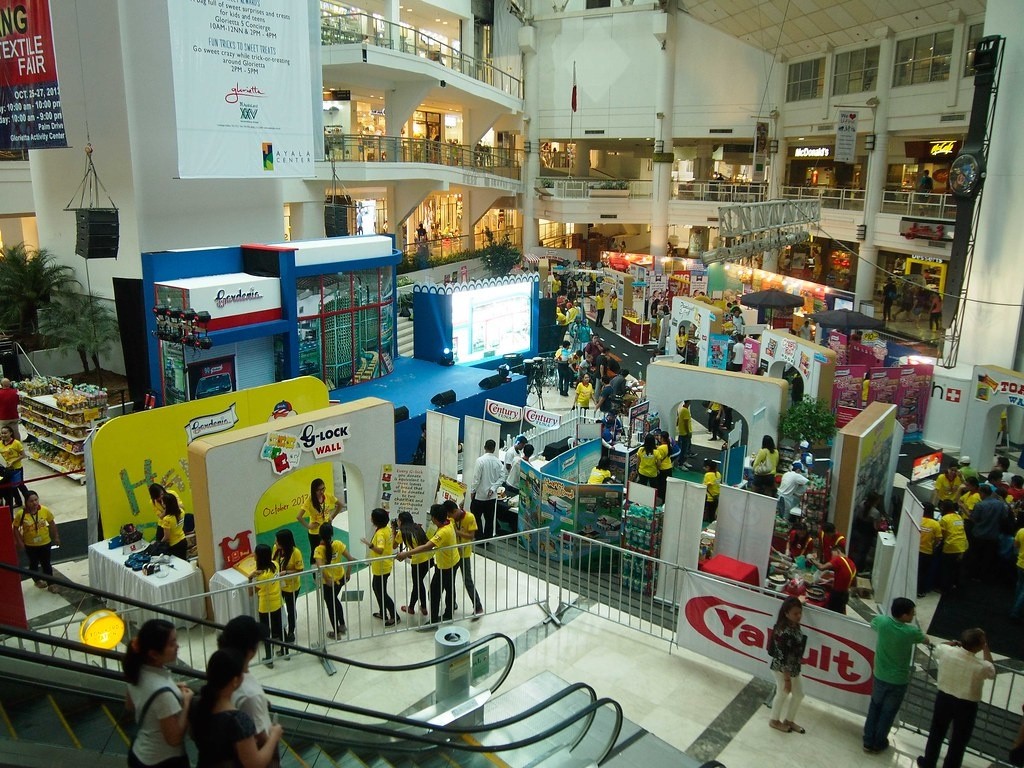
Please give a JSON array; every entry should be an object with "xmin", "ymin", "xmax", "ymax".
[
  {"xmin": 263, "ymin": 602, "xmax": 483, "ymax": 669},
  {"xmin": 784, "ymin": 719, "xmax": 805, "ymax": 734},
  {"xmin": 48, "ymin": 585, "xmax": 58, "ymax": 594},
  {"xmin": 560, "ymin": 389, "xmax": 569, "ymax": 396},
  {"xmin": 769, "ymin": 719, "xmax": 792, "ymax": 733},
  {"xmin": 677, "ymin": 459, "xmax": 692, "ymax": 471},
  {"xmin": 917, "ymin": 756, "xmax": 926, "ymax": 768},
  {"xmin": 36, "ymin": 580, "xmax": 47, "ymax": 588},
  {"xmin": 872, "ymin": 739, "xmax": 889, "ymax": 754},
  {"xmin": 708, "ymin": 437, "xmax": 717, "ymax": 441},
  {"xmin": 12, "ymin": 503, "xmax": 22, "ymax": 508},
  {"xmin": 864, "ymin": 742, "xmax": 872, "ymax": 751}
]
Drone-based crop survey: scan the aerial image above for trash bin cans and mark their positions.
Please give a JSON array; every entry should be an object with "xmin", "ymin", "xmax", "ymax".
[{"xmin": 434, "ymin": 626, "xmax": 470, "ymax": 703}]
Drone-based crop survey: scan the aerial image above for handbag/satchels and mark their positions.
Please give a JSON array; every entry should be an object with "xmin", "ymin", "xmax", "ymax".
[
  {"xmin": 754, "ymin": 456, "xmax": 772, "ymax": 474},
  {"xmin": 769, "ymin": 624, "xmax": 807, "ymax": 658}
]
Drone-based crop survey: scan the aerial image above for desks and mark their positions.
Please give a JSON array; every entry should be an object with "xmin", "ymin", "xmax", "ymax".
[
  {"xmin": 872, "ymin": 530, "xmax": 895, "ymax": 604},
  {"xmin": 88, "ymin": 535, "xmax": 208, "ymax": 629},
  {"xmin": 498, "ymin": 490, "xmax": 519, "ymax": 522},
  {"xmin": 701, "ymin": 553, "xmax": 760, "ymax": 594},
  {"xmin": 209, "ymin": 567, "xmax": 288, "ymax": 628}
]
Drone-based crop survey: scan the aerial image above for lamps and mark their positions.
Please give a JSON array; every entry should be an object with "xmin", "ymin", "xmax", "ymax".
[
  {"xmin": 698, "ymin": 222, "xmax": 809, "ymax": 268},
  {"xmin": 152, "ymin": 305, "xmax": 212, "ymax": 350},
  {"xmin": 441, "ymin": 349, "xmax": 453, "ymax": 366},
  {"xmin": 655, "ymin": 140, "xmax": 664, "ymax": 153},
  {"xmin": 856, "ymin": 225, "xmax": 866, "ymax": 239},
  {"xmin": 522, "ymin": 357, "xmax": 543, "ymax": 384},
  {"xmin": 498, "ymin": 363, "xmax": 510, "ymax": 377},
  {"xmin": 864, "ymin": 134, "xmax": 875, "ymax": 150},
  {"xmin": 769, "ymin": 140, "xmax": 778, "ymax": 153},
  {"xmin": 523, "ymin": 141, "xmax": 530, "ymax": 153}
]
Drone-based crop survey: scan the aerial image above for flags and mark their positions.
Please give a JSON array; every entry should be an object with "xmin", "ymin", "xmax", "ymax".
[{"xmin": 572, "ymin": 86, "xmax": 577, "ymax": 112}]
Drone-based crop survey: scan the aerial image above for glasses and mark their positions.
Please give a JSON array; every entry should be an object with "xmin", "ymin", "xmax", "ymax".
[{"xmin": 316, "ymin": 487, "xmax": 326, "ymax": 492}]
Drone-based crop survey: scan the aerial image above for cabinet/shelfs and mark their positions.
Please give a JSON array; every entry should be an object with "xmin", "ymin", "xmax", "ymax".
[
  {"xmin": 324, "ymin": 109, "xmax": 342, "ymax": 127},
  {"xmin": 619, "ymin": 480, "xmax": 664, "ymax": 597},
  {"xmin": 802, "ymin": 469, "xmax": 831, "ymax": 562},
  {"xmin": 17, "ymin": 390, "xmax": 111, "ymax": 485}
]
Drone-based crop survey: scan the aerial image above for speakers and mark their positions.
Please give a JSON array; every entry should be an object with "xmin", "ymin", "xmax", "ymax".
[
  {"xmin": 478, "ymin": 374, "xmax": 502, "ymax": 388},
  {"xmin": 75, "ymin": 210, "xmax": 120, "ymax": 259},
  {"xmin": 324, "ymin": 205, "xmax": 352, "ymax": 237},
  {"xmin": 430, "ymin": 390, "xmax": 456, "ymax": 404},
  {"xmin": 393, "ymin": 406, "xmax": 409, "ymax": 424}
]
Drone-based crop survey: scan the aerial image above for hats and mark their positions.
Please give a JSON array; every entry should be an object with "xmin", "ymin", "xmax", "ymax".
[
  {"xmin": 793, "ymin": 463, "xmax": 806, "ymax": 473},
  {"xmin": 885, "ymin": 277, "xmax": 895, "ymax": 283},
  {"xmin": 958, "ymin": 456, "xmax": 970, "ymax": 466},
  {"xmin": 567, "ymin": 303, "xmax": 572, "ymax": 307},
  {"xmin": 517, "ymin": 436, "xmax": 528, "ymax": 445}
]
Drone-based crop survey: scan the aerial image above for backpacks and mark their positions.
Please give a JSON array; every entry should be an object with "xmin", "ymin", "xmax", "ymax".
[{"xmin": 925, "ymin": 177, "xmax": 933, "ymax": 189}]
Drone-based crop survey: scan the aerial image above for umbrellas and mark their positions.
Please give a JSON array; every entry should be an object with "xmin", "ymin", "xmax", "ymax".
[
  {"xmin": 739, "ymin": 288, "xmax": 804, "ymax": 330},
  {"xmin": 804, "ymin": 307, "xmax": 885, "ymax": 335}
]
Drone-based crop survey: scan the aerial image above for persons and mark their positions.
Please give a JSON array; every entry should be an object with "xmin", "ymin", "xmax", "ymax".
[
  {"xmin": 119, "ymin": 614, "xmax": 283, "ymax": 768},
  {"xmin": 13, "ymin": 490, "xmax": 61, "ymax": 593},
  {"xmin": 717, "ymin": 174, "xmax": 724, "ymax": 184},
  {"xmin": 540, "ymin": 143, "xmax": 557, "ymax": 166},
  {"xmin": 559, "ymin": 238, "xmax": 567, "ymax": 248},
  {"xmin": 918, "ymin": 169, "xmax": 933, "ymax": 205},
  {"xmin": 430, "ymin": 126, "xmax": 438, "ymax": 140},
  {"xmin": 783, "ymin": 250, "xmax": 944, "ymax": 332},
  {"xmin": 433, "ymin": 136, "xmax": 491, "ymax": 166},
  {"xmin": 248, "ymin": 478, "xmax": 484, "ymax": 669},
  {"xmin": 677, "ymin": 290, "xmax": 862, "ymax": 407},
  {"xmin": 418, "ymin": 223, "xmax": 428, "ymax": 247},
  {"xmin": 585, "ymin": 400, "xmax": 732, "ymax": 503},
  {"xmin": 149, "ymin": 483, "xmax": 187, "ymax": 561},
  {"xmin": 917, "ymin": 624, "xmax": 996, "ymax": 768},
  {"xmin": 862, "ymin": 597, "xmax": 930, "ymax": 755},
  {"xmin": 416, "ymin": 422, "xmax": 535, "ymax": 547},
  {"xmin": 0, "ymin": 426, "xmax": 29, "ymax": 505},
  {"xmin": 930, "ymin": 456, "xmax": 940, "ymax": 473},
  {"xmin": 552, "ymin": 259, "xmax": 669, "ymax": 412},
  {"xmin": 610, "ymin": 238, "xmax": 626, "ymax": 251},
  {"xmin": 521, "ymin": 266, "xmax": 538, "ymax": 273},
  {"xmin": 703, "ymin": 432, "xmax": 857, "ymax": 616},
  {"xmin": 769, "ymin": 597, "xmax": 808, "ymax": 734},
  {"xmin": 917, "ymin": 408, "xmax": 1024, "ymax": 597}
]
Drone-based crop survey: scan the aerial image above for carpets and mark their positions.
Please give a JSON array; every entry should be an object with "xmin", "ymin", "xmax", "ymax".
[{"xmin": 256, "ymin": 517, "xmax": 368, "ymax": 602}]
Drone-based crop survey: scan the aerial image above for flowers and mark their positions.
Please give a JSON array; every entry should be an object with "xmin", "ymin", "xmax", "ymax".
[
  {"xmin": 541, "ymin": 179, "xmax": 553, "ymax": 187},
  {"xmin": 588, "ymin": 179, "xmax": 628, "ymax": 190}
]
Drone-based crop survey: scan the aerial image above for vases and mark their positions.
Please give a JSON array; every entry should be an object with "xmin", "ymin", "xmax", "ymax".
[{"xmin": 588, "ymin": 189, "xmax": 631, "ymax": 197}]
